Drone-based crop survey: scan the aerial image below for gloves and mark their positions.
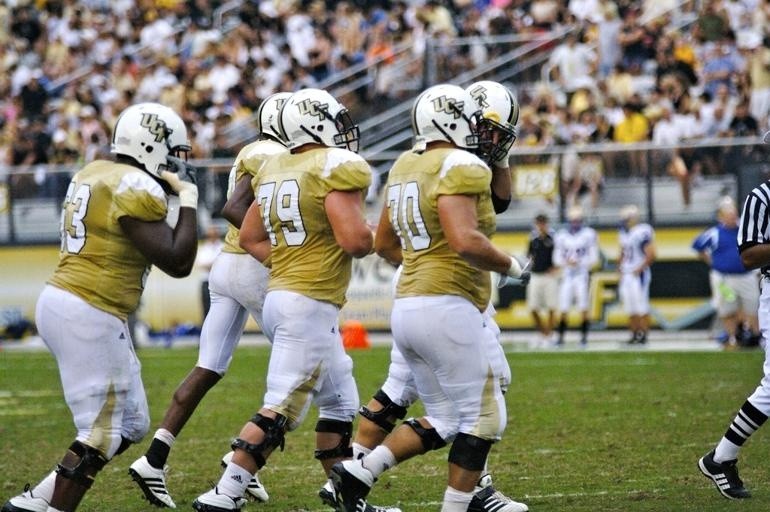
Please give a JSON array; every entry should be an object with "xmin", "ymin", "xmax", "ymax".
[{"xmin": 496, "ymin": 256, "xmax": 531, "ymax": 290}]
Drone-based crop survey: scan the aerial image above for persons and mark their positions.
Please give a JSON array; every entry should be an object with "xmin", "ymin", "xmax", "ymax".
[
  {"xmin": 2, "ymin": 101, "xmax": 198, "ymax": 511},
  {"xmin": 193, "ymin": 87, "xmax": 404, "ymax": 511},
  {"xmin": 616, "ymin": 205, "xmax": 656, "ymax": 345},
  {"xmin": 552, "ymin": 206, "xmax": 603, "ymax": 345},
  {"xmin": 525, "ymin": 216, "xmax": 558, "ymax": 345},
  {"xmin": 0, "ymin": 1, "xmax": 121, "ymax": 164},
  {"xmin": 693, "ymin": 197, "xmax": 761, "ymax": 351},
  {"xmin": 120, "ymin": 0, "xmax": 517, "ymax": 96},
  {"xmin": 518, "ymin": 1, "xmax": 770, "ymax": 207},
  {"xmin": 199, "ymin": 93, "xmax": 258, "ymax": 160},
  {"xmin": 129, "ymin": 91, "xmax": 294, "ymax": 509},
  {"xmin": 698, "ymin": 178, "xmax": 770, "ymax": 501}
]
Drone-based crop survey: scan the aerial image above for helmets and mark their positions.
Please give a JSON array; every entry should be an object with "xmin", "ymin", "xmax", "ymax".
[
  {"xmin": 256, "ymin": 91, "xmax": 293, "ymax": 147},
  {"xmin": 109, "ymin": 102, "xmax": 196, "ymax": 198},
  {"xmin": 277, "ymin": 88, "xmax": 361, "ymax": 156},
  {"xmin": 410, "ymin": 83, "xmax": 493, "ymax": 171},
  {"xmin": 464, "ymin": 79, "xmax": 520, "ymax": 164}
]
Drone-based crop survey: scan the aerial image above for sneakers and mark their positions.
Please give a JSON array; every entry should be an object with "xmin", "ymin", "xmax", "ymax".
[
  {"xmin": 3, "ymin": 482, "xmax": 49, "ymax": 512},
  {"xmin": 191, "ymin": 486, "xmax": 249, "ymax": 511},
  {"xmin": 318, "ymin": 478, "xmax": 340, "ymax": 511},
  {"xmin": 220, "ymin": 450, "xmax": 270, "ymax": 502},
  {"xmin": 128, "ymin": 455, "xmax": 177, "ymax": 509},
  {"xmin": 441, "ymin": 473, "xmax": 528, "ymax": 512},
  {"xmin": 329, "ymin": 457, "xmax": 376, "ymax": 511},
  {"xmin": 698, "ymin": 448, "xmax": 753, "ymax": 502},
  {"xmin": 156, "ymin": 155, "xmax": 199, "ymax": 210}
]
